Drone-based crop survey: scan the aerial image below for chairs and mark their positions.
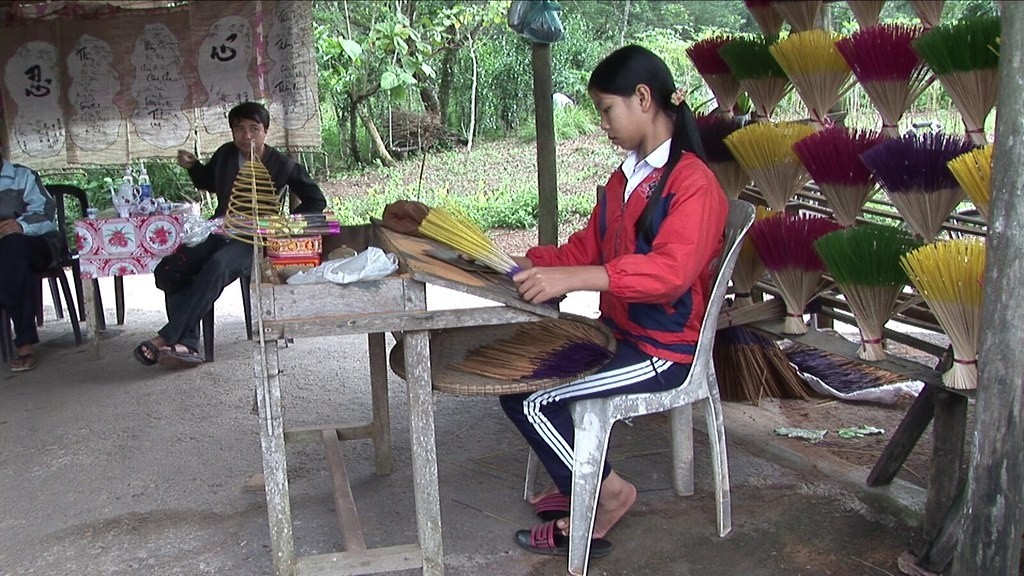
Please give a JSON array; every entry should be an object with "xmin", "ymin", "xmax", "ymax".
[
  {"xmin": 201, "ymin": 153, "xmax": 302, "ymax": 361},
  {"xmin": 525, "ymin": 199, "xmax": 756, "ymax": 573},
  {"xmin": 0, "ymin": 184, "xmax": 125, "ymax": 367}
]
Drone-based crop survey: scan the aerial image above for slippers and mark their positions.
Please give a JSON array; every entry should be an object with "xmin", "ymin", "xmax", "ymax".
[
  {"xmin": 11, "ymin": 353, "xmax": 39, "ymax": 372},
  {"xmin": 514, "ymin": 493, "xmax": 613, "ymax": 558}
]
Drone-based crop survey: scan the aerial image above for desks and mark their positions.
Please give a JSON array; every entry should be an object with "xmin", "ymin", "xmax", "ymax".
[{"xmin": 75, "ymin": 203, "xmax": 199, "ymax": 362}]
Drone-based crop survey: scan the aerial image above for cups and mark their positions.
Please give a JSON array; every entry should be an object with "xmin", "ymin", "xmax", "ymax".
[
  {"xmin": 138, "ymin": 197, "xmax": 171, "ymax": 215},
  {"xmin": 86, "ymin": 208, "xmax": 98, "ymax": 220}
]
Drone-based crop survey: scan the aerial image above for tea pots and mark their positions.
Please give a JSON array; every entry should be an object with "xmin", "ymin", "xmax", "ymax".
[{"xmin": 109, "ymin": 183, "xmax": 142, "ymax": 217}]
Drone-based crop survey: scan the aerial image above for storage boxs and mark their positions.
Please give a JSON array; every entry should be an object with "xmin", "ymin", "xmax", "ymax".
[{"xmin": 266, "ymin": 237, "xmax": 322, "ymax": 271}]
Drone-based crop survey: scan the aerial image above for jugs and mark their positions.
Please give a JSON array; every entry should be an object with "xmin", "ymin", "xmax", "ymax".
[{"xmin": 122, "ymin": 169, "xmax": 155, "ymax": 203}]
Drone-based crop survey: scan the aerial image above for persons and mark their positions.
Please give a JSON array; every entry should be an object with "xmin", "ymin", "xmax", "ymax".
[
  {"xmin": 133, "ymin": 101, "xmax": 327, "ymax": 368},
  {"xmin": 499, "ymin": 45, "xmax": 731, "ymax": 541},
  {"xmin": 0, "ymin": 154, "xmax": 65, "ymax": 372}
]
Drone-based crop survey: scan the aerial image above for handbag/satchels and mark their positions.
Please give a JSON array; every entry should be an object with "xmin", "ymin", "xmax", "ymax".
[{"xmin": 286, "ymin": 246, "xmax": 399, "ymax": 284}]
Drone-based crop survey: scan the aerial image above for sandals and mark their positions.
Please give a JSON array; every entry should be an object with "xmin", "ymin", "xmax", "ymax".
[{"xmin": 134, "ymin": 340, "xmax": 204, "ymax": 370}]
